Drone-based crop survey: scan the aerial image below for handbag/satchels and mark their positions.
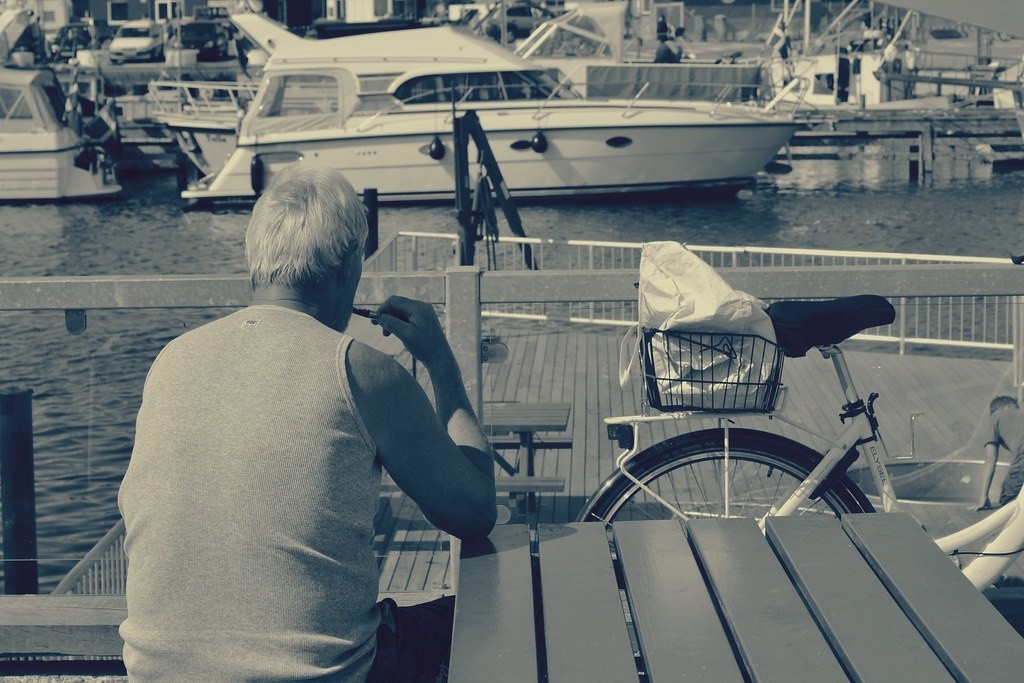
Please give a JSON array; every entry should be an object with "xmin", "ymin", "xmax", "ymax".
[{"xmin": 618, "ymin": 240, "xmax": 776, "ymax": 409}]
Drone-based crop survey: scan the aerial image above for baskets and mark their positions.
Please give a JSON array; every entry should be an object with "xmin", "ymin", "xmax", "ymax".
[{"xmin": 635, "ymin": 329, "xmax": 784, "ymax": 412}]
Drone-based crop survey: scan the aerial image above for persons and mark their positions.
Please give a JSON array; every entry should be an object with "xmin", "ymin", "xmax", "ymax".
[
  {"xmin": 655, "ymin": 35, "xmax": 684, "ymax": 64},
  {"xmin": 900, "ymin": 43, "xmax": 924, "ymax": 100},
  {"xmin": 116, "ymin": 161, "xmax": 493, "ymax": 682},
  {"xmin": 656, "ymin": 14, "xmax": 670, "ymax": 43}
]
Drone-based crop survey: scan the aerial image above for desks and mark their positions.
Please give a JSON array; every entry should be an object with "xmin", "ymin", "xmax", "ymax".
[
  {"xmin": 481, "ymin": 402, "xmax": 572, "ymax": 515},
  {"xmin": 447, "ymin": 514, "xmax": 1023, "ymax": 683}
]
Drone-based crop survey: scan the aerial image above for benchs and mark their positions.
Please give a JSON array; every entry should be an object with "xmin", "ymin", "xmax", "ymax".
[
  {"xmin": 485, "ymin": 435, "xmax": 572, "ymax": 453},
  {"xmin": 372, "ymin": 477, "xmax": 567, "ymax": 496}
]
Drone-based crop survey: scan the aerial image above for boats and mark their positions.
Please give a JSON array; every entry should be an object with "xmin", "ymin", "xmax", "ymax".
[
  {"xmin": 0, "ymin": 0, "xmax": 1024, "ymax": 202},
  {"xmin": 176, "ymin": 0, "xmax": 811, "ymax": 213}
]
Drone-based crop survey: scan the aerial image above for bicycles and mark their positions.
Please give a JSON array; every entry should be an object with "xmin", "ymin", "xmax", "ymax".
[{"xmin": 572, "ymin": 255, "xmax": 1024, "ymax": 682}]
